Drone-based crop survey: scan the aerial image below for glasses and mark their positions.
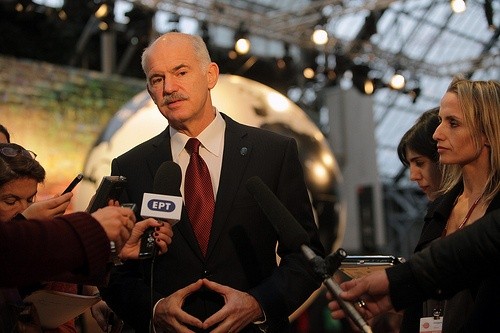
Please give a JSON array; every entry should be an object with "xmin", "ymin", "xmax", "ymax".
[{"xmin": 0, "ymin": 146, "xmax": 37, "ymax": 160}]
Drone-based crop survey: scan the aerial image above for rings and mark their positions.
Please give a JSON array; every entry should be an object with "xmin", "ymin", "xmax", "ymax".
[{"xmin": 358, "ymin": 300, "xmax": 366, "ymax": 308}]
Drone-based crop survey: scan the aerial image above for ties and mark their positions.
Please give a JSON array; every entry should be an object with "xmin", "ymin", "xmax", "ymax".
[{"xmin": 185, "ymin": 138, "xmax": 214, "ymax": 258}]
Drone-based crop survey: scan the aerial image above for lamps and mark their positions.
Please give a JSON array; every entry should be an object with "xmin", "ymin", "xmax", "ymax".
[{"xmin": 233, "ymin": 21, "xmax": 250, "ymax": 54}]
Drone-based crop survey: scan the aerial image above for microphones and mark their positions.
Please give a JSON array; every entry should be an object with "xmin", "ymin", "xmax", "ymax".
[
  {"xmin": 246, "ymin": 176, "xmax": 373, "ymax": 333},
  {"xmin": 140, "ymin": 161, "xmax": 184, "ymax": 266}
]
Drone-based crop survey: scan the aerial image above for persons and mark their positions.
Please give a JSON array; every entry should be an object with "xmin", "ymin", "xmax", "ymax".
[
  {"xmin": 325, "ymin": 78, "xmax": 500, "ymax": 333},
  {"xmin": 95, "ymin": 32, "xmax": 328, "ymax": 333},
  {"xmin": 0, "ymin": 125, "xmax": 174, "ymax": 333}
]
